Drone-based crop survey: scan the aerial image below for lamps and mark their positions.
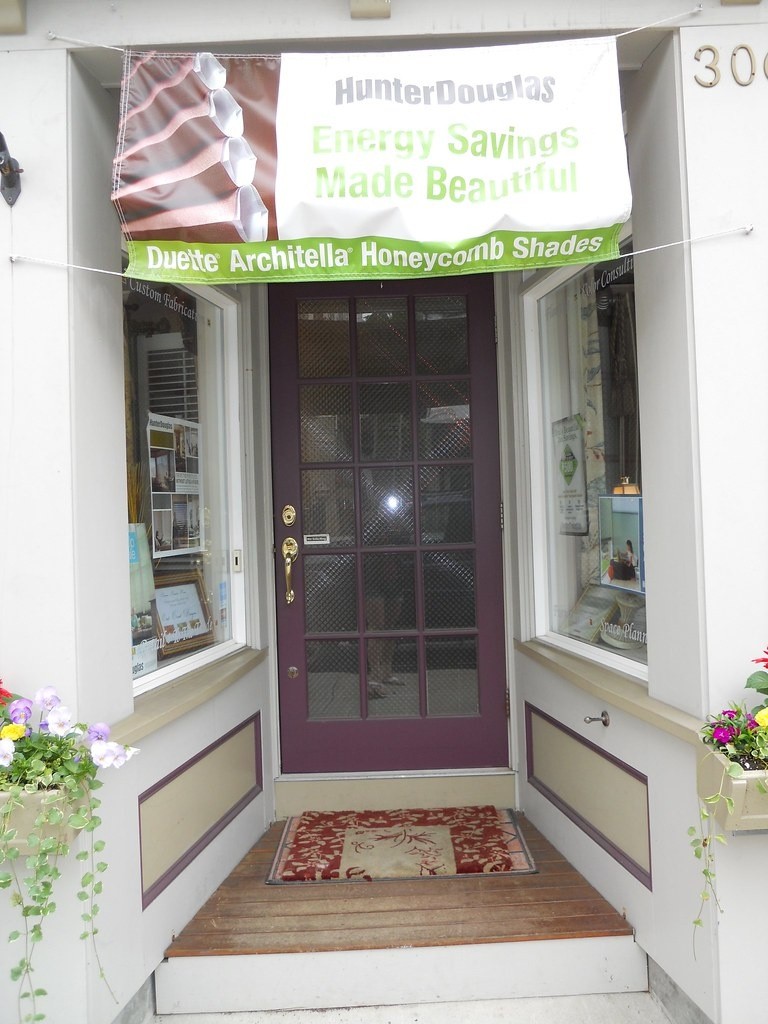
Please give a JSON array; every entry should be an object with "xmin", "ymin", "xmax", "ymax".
[{"xmin": 613, "ymin": 477, "xmax": 639, "ymax": 495}]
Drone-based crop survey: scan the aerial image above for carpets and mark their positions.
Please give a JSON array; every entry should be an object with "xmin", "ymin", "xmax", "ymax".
[{"xmin": 265, "ymin": 805, "xmax": 539, "ymax": 885}]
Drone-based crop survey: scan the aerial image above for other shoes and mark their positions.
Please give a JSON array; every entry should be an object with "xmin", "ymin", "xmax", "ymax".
[
  {"xmin": 367, "ymin": 679, "xmax": 386, "ymax": 697},
  {"xmin": 381, "ymin": 674, "xmax": 405, "ymax": 686}
]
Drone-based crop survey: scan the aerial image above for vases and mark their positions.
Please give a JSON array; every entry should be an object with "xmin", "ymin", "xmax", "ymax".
[
  {"xmin": 696, "ymin": 734, "xmax": 768, "ymax": 831},
  {"xmin": 0, "ymin": 780, "xmax": 91, "ymax": 855}
]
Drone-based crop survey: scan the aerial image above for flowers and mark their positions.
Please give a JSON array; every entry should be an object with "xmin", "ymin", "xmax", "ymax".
[
  {"xmin": 0, "ymin": 678, "xmax": 121, "ymax": 1024},
  {"xmin": 684, "ymin": 646, "xmax": 768, "ymax": 961}
]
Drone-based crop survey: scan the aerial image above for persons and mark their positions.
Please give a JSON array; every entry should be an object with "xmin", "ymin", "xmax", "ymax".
[
  {"xmin": 621, "ymin": 540, "xmax": 633, "ymax": 568},
  {"xmin": 156, "ymin": 530, "xmax": 170, "ymax": 546},
  {"xmin": 342, "ymin": 483, "xmax": 412, "ymax": 700}
]
